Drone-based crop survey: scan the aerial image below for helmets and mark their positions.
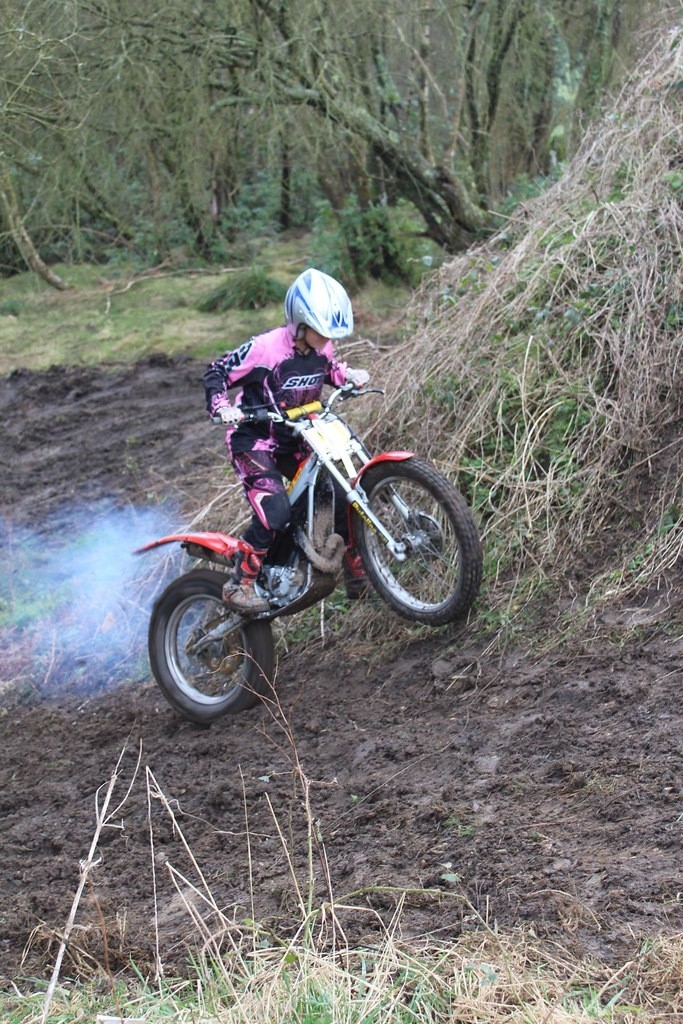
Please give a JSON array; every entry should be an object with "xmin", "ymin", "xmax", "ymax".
[{"xmin": 284, "ymin": 268, "xmax": 355, "ymax": 339}]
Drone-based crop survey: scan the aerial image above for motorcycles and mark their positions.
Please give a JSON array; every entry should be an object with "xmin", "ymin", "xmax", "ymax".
[{"xmin": 131, "ymin": 377, "xmax": 485, "ymax": 727}]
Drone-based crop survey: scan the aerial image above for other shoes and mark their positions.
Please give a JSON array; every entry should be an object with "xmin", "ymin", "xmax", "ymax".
[
  {"xmin": 346, "ymin": 584, "xmax": 382, "ymax": 601},
  {"xmin": 222, "ymin": 580, "xmax": 271, "ymax": 615}
]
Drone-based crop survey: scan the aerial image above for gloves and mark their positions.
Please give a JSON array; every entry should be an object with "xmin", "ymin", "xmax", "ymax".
[
  {"xmin": 216, "ymin": 408, "xmax": 246, "ymax": 426},
  {"xmin": 344, "ymin": 367, "xmax": 369, "ymax": 395}
]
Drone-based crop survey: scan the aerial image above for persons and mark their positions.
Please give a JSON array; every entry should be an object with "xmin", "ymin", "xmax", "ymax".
[{"xmin": 200, "ymin": 268, "xmax": 372, "ymax": 617}]
